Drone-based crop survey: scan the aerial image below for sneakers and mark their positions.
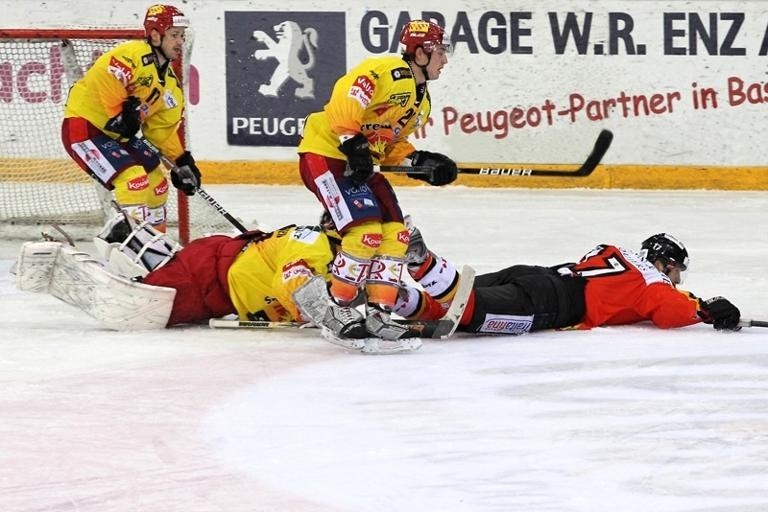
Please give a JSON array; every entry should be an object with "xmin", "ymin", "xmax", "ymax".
[
  {"xmin": 96, "ymin": 212, "xmax": 131, "ymax": 248},
  {"xmin": 9, "ymin": 234, "xmax": 63, "ymax": 277}
]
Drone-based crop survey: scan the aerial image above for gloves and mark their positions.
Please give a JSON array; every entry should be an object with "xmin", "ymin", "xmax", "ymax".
[
  {"xmin": 101, "ymin": 96, "xmax": 144, "ymax": 140},
  {"xmin": 406, "ymin": 150, "xmax": 457, "ymax": 187},
  {"xmin": 700, "ymin": 295, "xmax": 741, "ymax": 331},
  {"xmin": 339, "ymin": 131, "xmax": 374, "ymax": 188},
  {"xmin": 171, "ymin": 150, "xmax": 202, "ymax": 196}
]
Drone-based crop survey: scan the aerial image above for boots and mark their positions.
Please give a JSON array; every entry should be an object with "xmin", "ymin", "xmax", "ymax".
[
  {"xmin": 403, "ymin": 214, "xmax": 427, "ymax": 266},
  {"xmin": 322, "ymin": 299, "xmax": 421, "ymax": 341}
]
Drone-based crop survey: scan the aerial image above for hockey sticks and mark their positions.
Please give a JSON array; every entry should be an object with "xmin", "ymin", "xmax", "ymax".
[
  {"xmin": 346, "ymin": 130, "xmax": 613, "ymax": 177},
  {"xmin": 209, "ymin": 264, "xmax": 475, "ymax": 339}
]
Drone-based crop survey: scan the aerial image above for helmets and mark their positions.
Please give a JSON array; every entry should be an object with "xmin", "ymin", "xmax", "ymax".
[
  {"xmin": 640, "ymin": 232, "xmax": 688, "ymax": 271},
  {"xmin": 400, "ymin": 19, "xmax": 451, "ymax": 55},
  {"xmin": 143, "ymin": 3, "xmax": 190, "ymax": 38}
]
